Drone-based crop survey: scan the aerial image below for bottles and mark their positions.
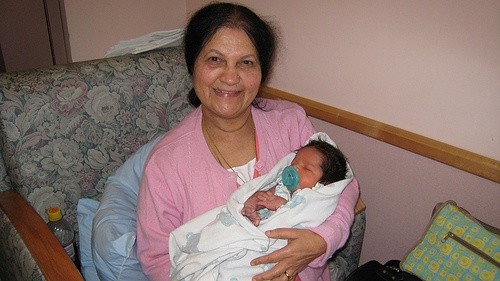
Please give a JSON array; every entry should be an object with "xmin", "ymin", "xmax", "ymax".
[{"xmin": 47, "ymin": 207, "xmax": 78, "ymax": 265}]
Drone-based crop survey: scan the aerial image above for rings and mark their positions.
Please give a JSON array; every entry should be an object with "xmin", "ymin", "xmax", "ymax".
[{"xmin": 284, "ymin": 271, "xmax": 290, "ymax": 278}]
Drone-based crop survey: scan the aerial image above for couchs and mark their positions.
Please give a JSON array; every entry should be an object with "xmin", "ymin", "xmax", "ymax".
[{"xmin": 0, "ymin": 46, "xmax": 367, "ymax": 281}]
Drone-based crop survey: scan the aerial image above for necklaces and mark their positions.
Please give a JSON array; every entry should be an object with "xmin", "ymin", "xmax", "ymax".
[{"xmin": 204, "ymin": 111, "xmax": 257, "ymax": 183}]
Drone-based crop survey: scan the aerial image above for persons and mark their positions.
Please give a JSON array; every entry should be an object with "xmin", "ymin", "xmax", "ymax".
[
  {"xmin": 168, "ymin": 131, "xmax": 354, "ymax": 281},
  {"xmin": 138, "ymin": 2, "xmax": 360, "ymax": 281}
]
